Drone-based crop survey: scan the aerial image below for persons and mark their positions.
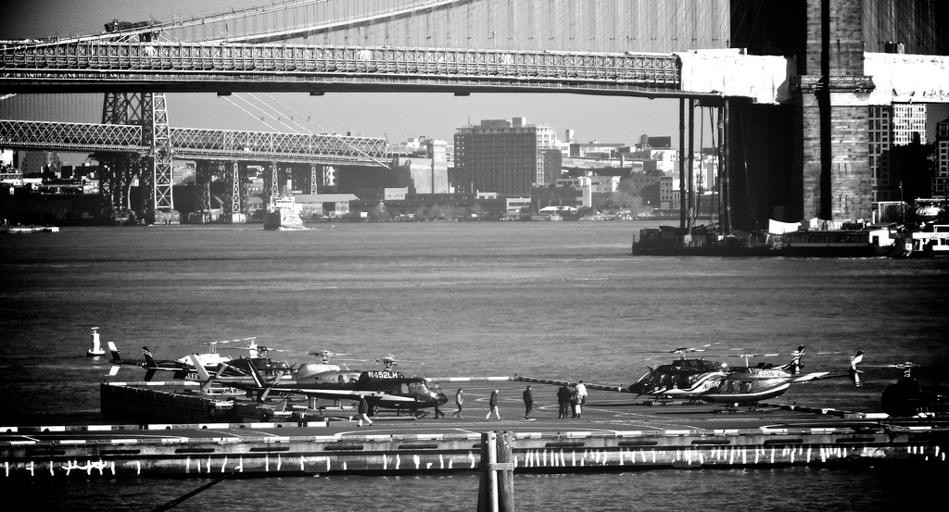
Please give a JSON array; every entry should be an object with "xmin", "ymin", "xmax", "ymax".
[
  {"xmin": 556, "ymin": 378, "xmax": 588, "ymax": 419},
  {"xmin": 433, "ymin": 405, "xmax": 446, "ymax": 419},
  {"xmin": 355, "ymin": 392, "xmax": 375, "ymax": 428},
  {"xmin": 522, "ymin": 384, "xmax": 536, "ymax": 420},
  {"xmin": 482, "ymin": 388, "xmax": 503, "ymax": 420},
  {"xmin": 451, "ymin": 387, "xmax": 465, "ymax": 420}
]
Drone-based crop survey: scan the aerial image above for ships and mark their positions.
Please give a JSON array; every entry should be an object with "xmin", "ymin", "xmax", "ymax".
[{"xmin": 263, "ymin": 183, "xmax": 303, "ymax": 230}]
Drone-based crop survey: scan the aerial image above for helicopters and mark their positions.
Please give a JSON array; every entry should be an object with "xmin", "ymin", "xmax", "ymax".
[{"xmin": 622, "ymin": 341, "xmax": 867, "ymax": 416}]
